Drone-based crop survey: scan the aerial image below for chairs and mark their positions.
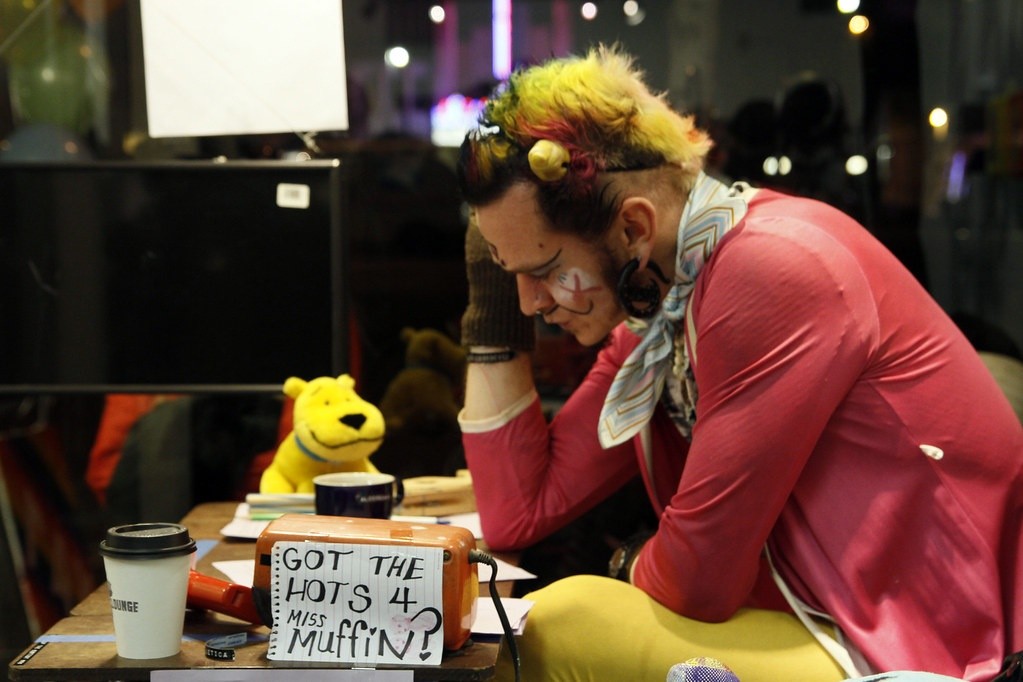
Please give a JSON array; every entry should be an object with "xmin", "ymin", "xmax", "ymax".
[{"xmin": 0, "ymin": 388, "xmax": 110, "ymax": 639}]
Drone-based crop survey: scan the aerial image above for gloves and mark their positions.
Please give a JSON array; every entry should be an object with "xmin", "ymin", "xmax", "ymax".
[
  {"xmin": 460, "ymin": 209, "xmax": 535, "ymax": 350},
  {"xmin": 608, "ymin": 529, "xmax": 655, "ymax": 582}
]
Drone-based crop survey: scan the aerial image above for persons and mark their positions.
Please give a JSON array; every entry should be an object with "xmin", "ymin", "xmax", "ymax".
[
  {"xmin": 457, "ymin": 42, "xmax": 1023, "ymax": 682},
  {"xmin": 721, "ymin": 69, "xmax": 865, "ymax": 213}
]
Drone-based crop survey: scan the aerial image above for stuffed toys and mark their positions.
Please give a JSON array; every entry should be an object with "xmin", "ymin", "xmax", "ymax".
[
  {"xmin": 382, "ymin": 330, "xmax": 466, "ymax": 477},
  {"xmin": 258, "ymin": 374, "xmax": 385, "ymax": 496}
]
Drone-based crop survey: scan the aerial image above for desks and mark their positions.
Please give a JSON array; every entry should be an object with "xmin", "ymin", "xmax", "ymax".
[{"xmin": 10, "ymin": 491, "xmax": 518, "ymax": 682}]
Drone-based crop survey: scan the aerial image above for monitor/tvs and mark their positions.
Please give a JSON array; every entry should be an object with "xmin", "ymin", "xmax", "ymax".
[{"xmin": 0, "ymin": 160, "xmax": 349, "ymax": 391}]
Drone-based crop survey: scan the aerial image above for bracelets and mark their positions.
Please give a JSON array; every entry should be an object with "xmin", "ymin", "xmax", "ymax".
[{"xmin": 465, "ymin": 349, "xmax": 516, "ymax": 364}]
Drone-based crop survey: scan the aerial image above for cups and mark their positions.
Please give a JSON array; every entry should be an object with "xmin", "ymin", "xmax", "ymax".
[
  {"xmin": 98, "ymin": 522, "xmax": 198, "ymax": 660},
  {"xmin": 311, "ymin": 472, "xmax": 396, "ymax": 519}
]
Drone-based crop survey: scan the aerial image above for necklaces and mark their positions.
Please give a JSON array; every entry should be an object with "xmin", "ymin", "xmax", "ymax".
[{"xmin": 668, "ymin": 334, "xmax": 684, "ymax": 376}]
